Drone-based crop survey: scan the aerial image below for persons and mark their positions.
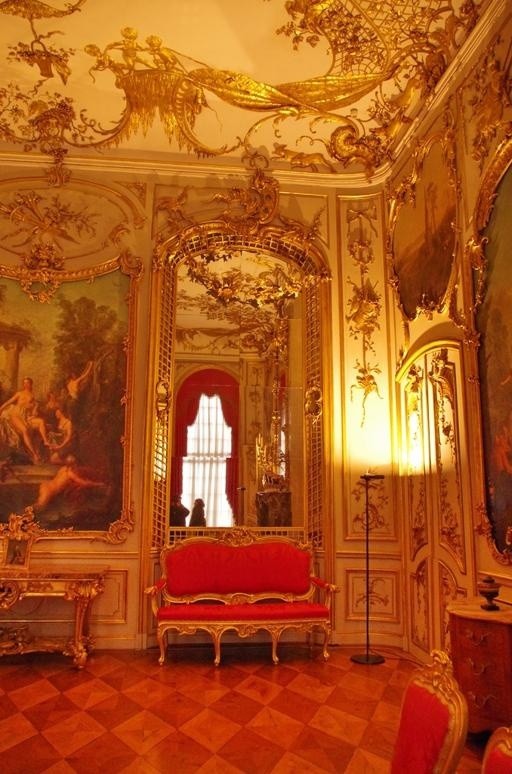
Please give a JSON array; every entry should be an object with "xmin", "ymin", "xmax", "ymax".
[
  {"xmin": 190, "ymin": 499, "xmax": 206, "ymax": 528},
  {"xmin": 170, "ymin": 496, "xmax": 188, "ymax": 528},
  {"xmin": 0, "ymin": 361, "xmax": 108, "ymax": 523}
]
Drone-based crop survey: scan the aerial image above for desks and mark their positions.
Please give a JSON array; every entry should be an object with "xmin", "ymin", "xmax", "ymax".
[
  {"xmin": 0, "ymin": 562, "xmax": 106, "ymax": 671},
  {"xmin": 440, "ymin": 598, "xmax": 512, "ymax": 731}
]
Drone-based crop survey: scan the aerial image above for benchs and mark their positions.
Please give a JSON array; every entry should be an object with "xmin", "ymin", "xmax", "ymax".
[{"xmin": 143, "ymin": 535, "xmax": 340, "ymax": 666}]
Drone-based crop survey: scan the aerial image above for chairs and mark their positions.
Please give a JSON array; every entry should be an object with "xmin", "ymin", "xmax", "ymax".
[
  {"xmin": 477, "ymin": 723, "xmax": 512, "ymax": 774},
  {"xmin": 392, "ymin": 649, "xmax": 471, "ymax": 773}
]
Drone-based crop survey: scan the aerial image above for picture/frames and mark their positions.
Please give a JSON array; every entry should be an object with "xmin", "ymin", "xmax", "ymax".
[
  {"xmin": 0, "ymin": 246, "xmax": 144, "ymax": 543},
  {"xmin": 465, "ymin": 137, "xmax": 512, "ymax": 568},
  {"xmin": 0, "ymin": 504, "xmax": 47, "ymax": 573}
]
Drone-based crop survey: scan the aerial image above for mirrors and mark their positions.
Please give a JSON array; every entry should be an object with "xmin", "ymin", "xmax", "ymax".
[{"xmin": 169, "ymin": 248, "xmax": 307, "ymax": 544}]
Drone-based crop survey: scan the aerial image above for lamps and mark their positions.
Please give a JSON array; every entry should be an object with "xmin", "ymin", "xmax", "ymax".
[{"xmin": 351, "ymin": 471, "xmax": 384, "ymax": 665}]
